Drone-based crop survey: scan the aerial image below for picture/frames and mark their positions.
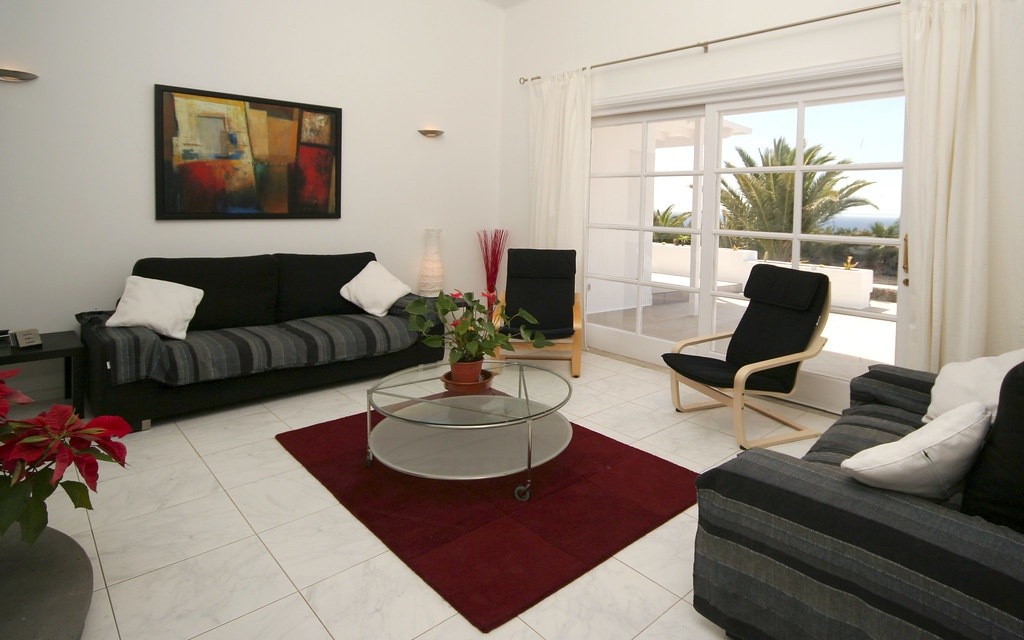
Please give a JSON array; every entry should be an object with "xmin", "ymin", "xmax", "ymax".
[{"xmin": 154, "ymin": 85, "xmax": 342, "ymax": 219}]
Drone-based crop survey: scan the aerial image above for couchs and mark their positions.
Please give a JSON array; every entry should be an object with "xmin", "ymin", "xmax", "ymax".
[
  {"xmin": 75, "ymin": 251, "xmax": 444, "ymax": 429},
  {"xmin": 691, "ymin": 362, "xmax": 1024, "ymax": 640}
]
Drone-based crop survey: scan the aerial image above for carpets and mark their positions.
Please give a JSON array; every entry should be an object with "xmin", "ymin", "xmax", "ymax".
[{"xmin": 275, "ymin": 387, "xmax": 700, "ymax": 633}]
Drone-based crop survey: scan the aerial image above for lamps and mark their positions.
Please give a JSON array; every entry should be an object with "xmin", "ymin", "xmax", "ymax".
[
  {"xmin": 417, "ymin": 122, "xmax": 445, "ymax": 137},
  {"xmin": 0, "ymin": 58, "xmax": 39, "ymax": 84}
]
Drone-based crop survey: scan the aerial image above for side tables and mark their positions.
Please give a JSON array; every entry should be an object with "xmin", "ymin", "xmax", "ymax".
[
  {"xmin": 0, "ymin": 329, "xmax": 87, "ymax": 420},
  {"xmin": 422, "ymin": 297, "xmax": 474, "ymax": 313}
]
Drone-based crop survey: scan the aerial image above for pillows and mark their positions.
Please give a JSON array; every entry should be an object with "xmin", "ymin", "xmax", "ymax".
[
  {"xmin": 921, "ymin": 347, "xmax": 1024, "ymax": 425},
  {"xmin": 840, "ymin": 400, "xmax": 993, "ymax": 499},
  {"xmin": 340, "ymin": 261, "xmax": 412, "ymax": 317},
  {"xmin": 961, "ymin": 362, "xmax": 1024, "ymax": 532},
  {"xmin": 105, "ymin": 275, "xmax": 204, "ymax": 341}
]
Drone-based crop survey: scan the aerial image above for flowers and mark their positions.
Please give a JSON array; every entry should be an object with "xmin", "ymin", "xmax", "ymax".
[
  {"xmin": 0, "ymin": 368, "xmax": 134, "ymax": 542},
  {"xmin": 402, "ymin": 288, "xmax": 556, "ymax": 367}
]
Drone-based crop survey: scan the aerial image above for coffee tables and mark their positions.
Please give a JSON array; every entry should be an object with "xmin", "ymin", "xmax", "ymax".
[{"xmin": 366, "ymin": 360, "xmax": 573, "ymax": 501}]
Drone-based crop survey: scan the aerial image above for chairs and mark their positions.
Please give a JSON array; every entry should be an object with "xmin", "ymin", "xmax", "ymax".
[
  {"xmin": 660, "ymin": 263, "xmax": 831, "ymax": 450},
  {"xmin": 492, "ymin": 248, "xmax": 584, "ymax": 378}
]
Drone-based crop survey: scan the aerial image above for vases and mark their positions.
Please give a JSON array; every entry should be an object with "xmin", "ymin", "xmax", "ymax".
[
  {"xmin": 0, "ymin": 521, "xmax": 94, "ymax": 640},
  {"xmin": 450, "ymin": 356, "xmax": 485, "ymax": 383}
]
